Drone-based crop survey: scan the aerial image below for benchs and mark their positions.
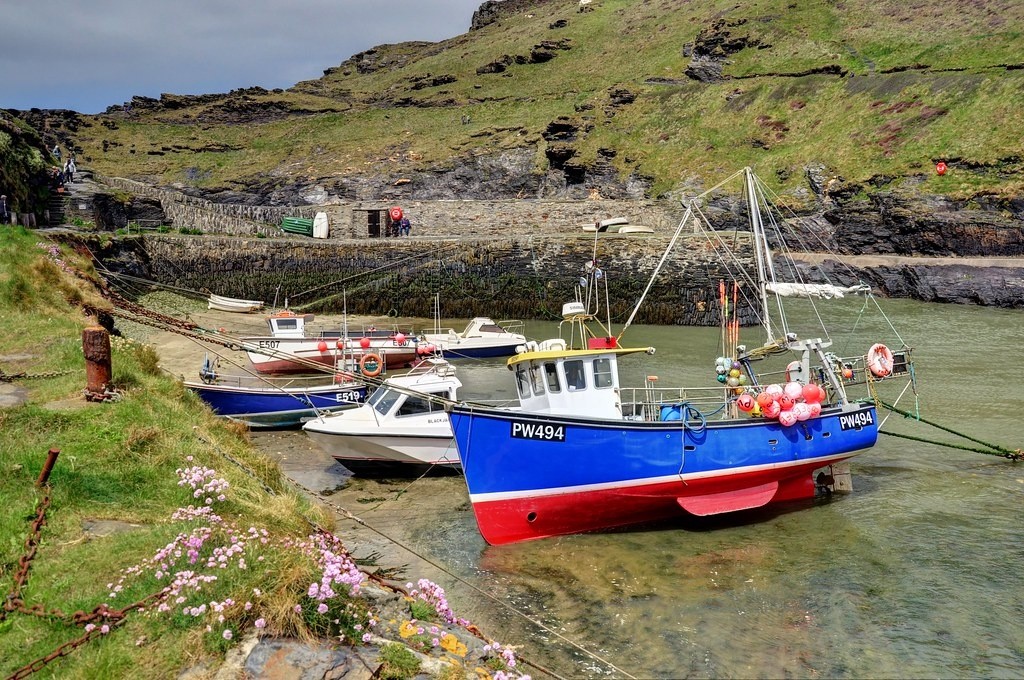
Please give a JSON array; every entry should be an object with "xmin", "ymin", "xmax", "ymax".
[{"xmin": 128, "ymin": 219, "xmax": 162, "ymax": 234}]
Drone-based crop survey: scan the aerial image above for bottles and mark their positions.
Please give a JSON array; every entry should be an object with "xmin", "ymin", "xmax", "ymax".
[{"xmin": 838, "ymin": 398, "xmax": 843, "ymax": 407}]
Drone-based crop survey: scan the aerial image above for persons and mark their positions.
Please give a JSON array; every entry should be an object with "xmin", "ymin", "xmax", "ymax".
[
  {"xmin": 391, "ymin": 216, "xmax": 410, "ymax": 237},
  {"xmin": 66, "ymin": 147, "xmax": 75, "ymax": 164},
  {"xmin": 52, "ymin": 166, "xmax": 64, "ymax": 186},
  {"xmin": 54, "ymin": 145, "xmax": 63, "ymax": 162},
  {"xmin": 64, "ymin": 158, "xmax": 76, "ymax": 183}
]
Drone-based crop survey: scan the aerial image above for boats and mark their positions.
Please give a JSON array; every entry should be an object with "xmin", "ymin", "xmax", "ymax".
[
  {"xmin": 297, "ymin": 350, "xmax": 649, "ymax": 479},
  {"xmin": 236, "ymin": 280, "xmax": 425, "ymax": 376},
  {"xmin": 282, "ymin": 218, "xmax": 313, "ymax": 235},
  {"xmin": 441, "ymin": 161, "xmax": 919, "ymax": 547},
  {"xmin": 313, "ymin": 212, "xmax": 329, "ymax": 238},
  {"xmin": 412, "ymin": 290, "xmax": 529, "ymax": 358},
  {"xmin": 181, "ymin": 287, "xmax": 372, "ymax": 431}
]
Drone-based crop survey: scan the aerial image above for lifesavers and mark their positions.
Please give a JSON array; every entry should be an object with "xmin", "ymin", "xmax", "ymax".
[
  {"xmin": 867, "ymin": 342, "xmax": 895, "ymax": 377},
  {"xmin": 359, "ymin": 352, "xmax": 383, "ymax": 377},
  {"xmin": 785, "ymin": 360, "xmax": 814, "ymax": 384}
]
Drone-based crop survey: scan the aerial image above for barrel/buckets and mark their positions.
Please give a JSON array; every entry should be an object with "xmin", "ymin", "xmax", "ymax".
[
  {"xmin": 8, "ymin": 211, "xmax": 17, "ymax": 227},
  {"xmin": 44, "ymin": 210, "xmax": 49, "ymax": 221},
  {"xmin": 20, "ymin": 213, "xmax": 29, "ymax": 228},
  {"xmin": 660, "ymin": 404, "xmax": 688, "ymax": 421},
  {"xmin": 29, "ymin": 213, "xmax": 36, "ymax": 228}
]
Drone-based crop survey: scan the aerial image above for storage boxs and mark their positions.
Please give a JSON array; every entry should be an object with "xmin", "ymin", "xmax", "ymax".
[{"xmin": 588, "ymin": 337, "xmax": 616, "ymax": 350}]
